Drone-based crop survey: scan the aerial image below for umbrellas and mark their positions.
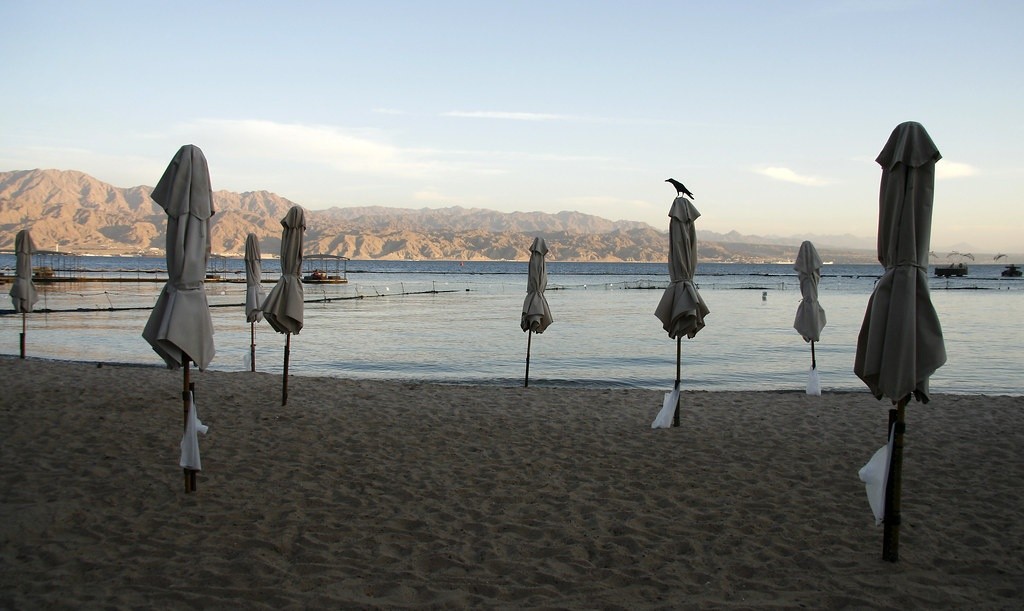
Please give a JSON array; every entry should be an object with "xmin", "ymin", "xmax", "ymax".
[
  {"xmin": 10, "ymin": 230, "xmax": 37, "ymax": 357},
  {"xmin": 655, "ymin": 197, "xmax": 711, "ymax": 426},
  {"xmin": 520, "ymin": 238, "xmax": 553, "ymax": 387},
  {"xmin": 142, "ymin": 145, "xmax": 215, "ymax": 491},
  {"xmin": 795, "ymin": 240, "xmax": 826, "ymax": 370},
  {"xmin": 244, "ymin": 233, "xmax": 266, "ymax": 372},
  {"xmin": 261, "ymin": 206, "xmax": 306, "ymax": 406},
  {"xmin": 854, "ymin": 122, "xmax": 946, "ymax": 562}
]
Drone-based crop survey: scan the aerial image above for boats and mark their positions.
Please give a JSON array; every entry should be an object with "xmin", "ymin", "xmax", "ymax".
[
  {"xmin": 301, "ymin": 255, "xmax": 351, "ymax": 284},
  {"xmin": 1001, "ymin": 264, "xmax": 1023, "ymax": 277}
]
